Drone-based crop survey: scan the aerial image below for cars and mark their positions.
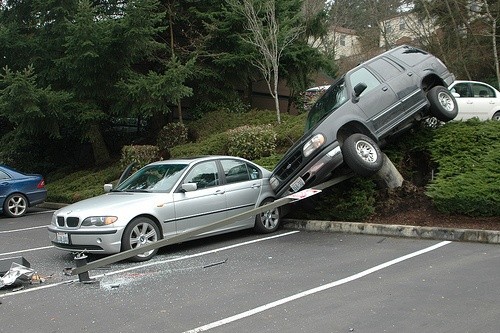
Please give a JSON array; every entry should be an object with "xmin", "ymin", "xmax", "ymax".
[
  {"xmin": 0, "ymin": 164, "xmax": 48, "ymax": 218},
  {"xmin": 445, "ymin": 80, "xmax": 500, "ymax": 124},
  {"xmin": 46, "ymin": 160, "xmax": 283, "ymax": 263}
]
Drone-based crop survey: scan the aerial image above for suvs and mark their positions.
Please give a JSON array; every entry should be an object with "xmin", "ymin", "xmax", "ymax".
[{"xmin": 269, "ymin": 43, "xmax": 457, "ymax": 200}]
